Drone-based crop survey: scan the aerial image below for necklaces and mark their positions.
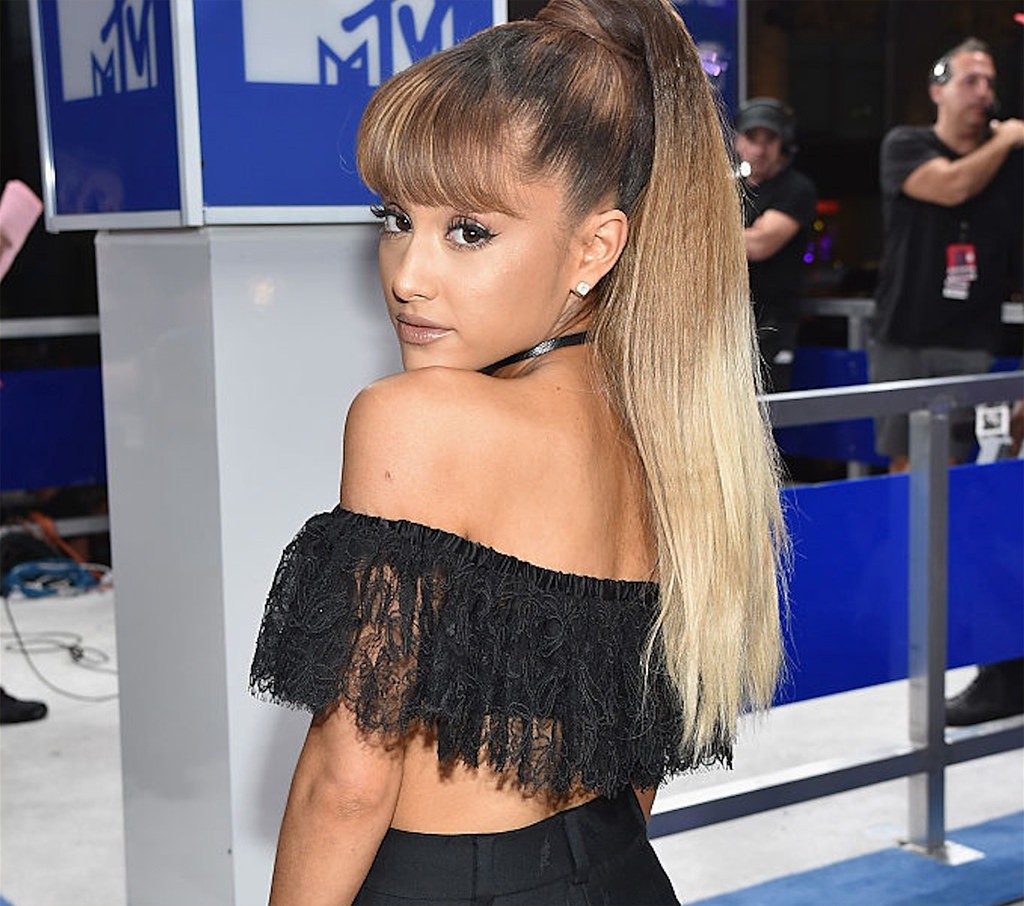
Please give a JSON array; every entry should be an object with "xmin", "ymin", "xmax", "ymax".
[{"xmin": 453, "ymin": 329, "xmax": 589, "ymax": 371}]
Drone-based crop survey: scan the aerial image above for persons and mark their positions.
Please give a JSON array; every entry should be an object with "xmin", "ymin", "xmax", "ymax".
[
  {"xmin": 735, "ymin": 38, "xmax": 1024, "ymax": 728},
  {"xmin": 0, "ymin": 182, "xmax": 48, "ymax": 724},
  {"xmin": 248, "ymin": 0, "xmax": 791, "ymax": 906}
]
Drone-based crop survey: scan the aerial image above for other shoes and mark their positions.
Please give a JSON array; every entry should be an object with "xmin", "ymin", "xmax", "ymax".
[
  {"xmin": 0, "ymin": 689, "xmax": 47, "ymax": 723},
  {"xmin": 945, "ymin": 672, "xmax": 1024, "ymax": 727}
]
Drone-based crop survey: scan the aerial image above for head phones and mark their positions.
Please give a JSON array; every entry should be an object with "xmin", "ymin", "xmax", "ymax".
[{"xmin": 730, "ymin": 98, "xmax": 800, "ymax": 161}]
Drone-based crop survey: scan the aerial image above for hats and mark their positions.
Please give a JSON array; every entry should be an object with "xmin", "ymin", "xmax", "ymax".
[{"xmin": 738, "ymin": 99, "xmax": 788, "ymax": 136}]
